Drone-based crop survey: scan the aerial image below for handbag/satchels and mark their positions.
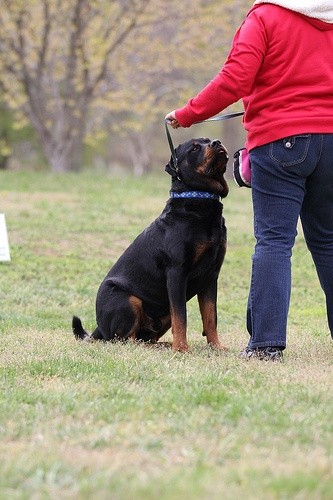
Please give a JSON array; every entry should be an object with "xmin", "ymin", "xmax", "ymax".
[{"xmin": 233, "ymin": 148, "xmax": 252, "ymax": 189}]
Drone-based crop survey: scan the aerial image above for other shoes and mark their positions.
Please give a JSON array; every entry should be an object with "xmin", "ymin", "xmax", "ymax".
[{"xmin": 239, "ymin": 346, "xmax": 282, "ymax": 364}]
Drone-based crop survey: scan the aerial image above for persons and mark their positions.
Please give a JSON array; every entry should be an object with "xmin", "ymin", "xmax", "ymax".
[{"xmin": 165, "ymin": 0, "xmax": 333, "ymax": 362}]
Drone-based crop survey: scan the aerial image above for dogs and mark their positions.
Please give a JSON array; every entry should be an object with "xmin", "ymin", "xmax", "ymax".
[{"xmin": 69, "ymin": 137, "xmax": 230, "ymax": 352}]
